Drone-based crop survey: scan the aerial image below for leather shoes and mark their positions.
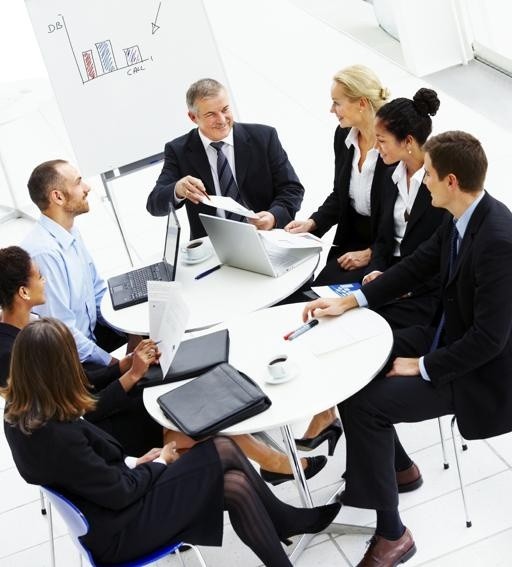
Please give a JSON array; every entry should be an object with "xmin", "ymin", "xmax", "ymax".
[
  {"xmin": 356, "ymin": 527, "xmax": 416, "ymax": 567},
  {"xmin": 342, "ymin": 462, "xmax": 423, "ymax": 493},
  {"xmin": 259, "ymin": 456, "xmax": 328, "ymax": 486}
]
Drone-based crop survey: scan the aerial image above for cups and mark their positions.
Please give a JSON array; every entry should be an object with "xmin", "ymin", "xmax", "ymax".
[
  {"xmin": 266, "ymin": 355, "xmax": 291, "ymax": 378},
  {"xmin": 182, "ymin": 240, "xmax": 206, "ymax": 258}
]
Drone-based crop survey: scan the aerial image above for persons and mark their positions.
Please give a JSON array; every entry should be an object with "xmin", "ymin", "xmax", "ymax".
[
  {"xmin": 146, "ymin": 79, "xmax": 305, "ymax": 241},
  {"xmin": 0, "ymin": 318, "xmax": 341, "ymax": 567},
  {"xmin": 19, "ymin": 160, "xmax": 149, "ymax": 370},
  {"xmin": 0, "ymin": 245, "xmax": 327, "ymax": 486},
  {"xmin": 294, "ymin": 88, "xmax": 445, "ymax": 456},
  {"xmin": 302, "ymin": 130, "xmax": 512, "ymax": 567},
  {"xmin": 284, "ymin": 66, "xmax": 391, "ymax": 302}
]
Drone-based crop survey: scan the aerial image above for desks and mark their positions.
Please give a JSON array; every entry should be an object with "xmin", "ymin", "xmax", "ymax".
[
  {"xmin": 100, "ymin": 230, "xmax": 326, "ymax": 343},
  {"xmin": 135, "ymin": 296, "xmax": 395, "ymax": 565}
]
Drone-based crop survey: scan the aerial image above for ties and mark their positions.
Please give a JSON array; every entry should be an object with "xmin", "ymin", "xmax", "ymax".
[
  {"xmin": 432, "ymin": 224, "xmax": 458, "ymax": 351},
  {"xmin": 211, "ymin": 140, "xmax": 249, "ymax": 223}
]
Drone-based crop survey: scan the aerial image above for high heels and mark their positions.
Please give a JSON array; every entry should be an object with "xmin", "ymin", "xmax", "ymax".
[
  {"xmin": 278, "ymin": 501, "xmax": 342, "ymax": 546},
  {"xmin": 283, "ymin": 420, "xmax": 344, "ymax": 455}
]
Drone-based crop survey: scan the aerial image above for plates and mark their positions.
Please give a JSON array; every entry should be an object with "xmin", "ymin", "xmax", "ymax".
[
  {"xmin": 181, "ymin": 250, "xmax": 213, "ymax": 265},
  {"xmin": 262, "ymin": 369, "xmax": 297, "ymax": 384}
]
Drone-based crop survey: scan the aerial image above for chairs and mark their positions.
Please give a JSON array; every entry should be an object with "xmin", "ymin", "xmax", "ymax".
[{"xmin": 37, "ymin": 485, "xmax": 212, "ymax": 567}]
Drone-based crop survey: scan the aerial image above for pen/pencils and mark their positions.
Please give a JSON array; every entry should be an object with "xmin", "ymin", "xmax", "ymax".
[
  {"xmin": 284, "ymin": 319, "xmax": 318, "ymax": 340},
  {"xmin": 197, "ymin": 186, "xmax": 211, "ymax": 200},
  {"xmin": 195, "ymin": 263, "xmax": 225, "ymax": 278},
  {"xmin": 127, "ymin": 339, "xmax": 162, "ymax": 357}
]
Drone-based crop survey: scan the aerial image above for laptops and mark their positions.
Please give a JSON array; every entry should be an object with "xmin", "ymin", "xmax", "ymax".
[
  {"xmin": 106, "ymin": 200, "xmax": 181, "ymax": 311},
  {"xmin": 199, "ymin": 213, "xmax": 322, "ymax": 277}
]
